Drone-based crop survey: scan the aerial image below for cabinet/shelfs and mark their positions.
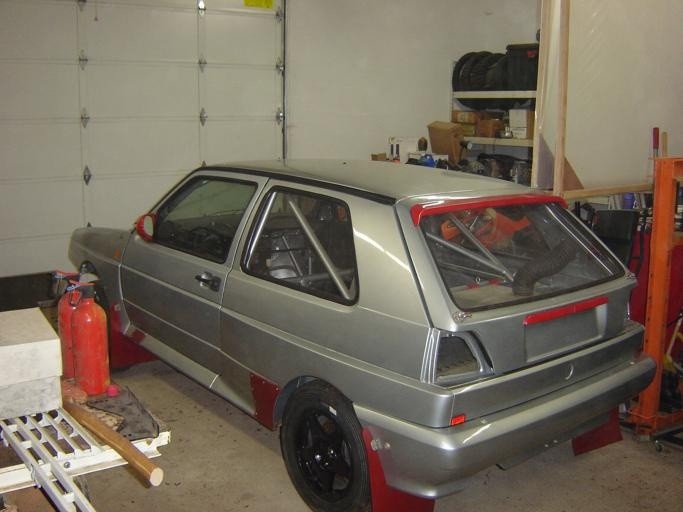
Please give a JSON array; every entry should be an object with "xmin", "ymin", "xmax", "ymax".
[{"xmin": 449, "ymin": 87, "xmax": 539, "ymax": 189}]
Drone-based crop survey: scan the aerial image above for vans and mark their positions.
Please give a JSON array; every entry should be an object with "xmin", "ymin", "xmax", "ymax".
[{"xmin": 69, "ymin": 159, "xmax": 656, "ymax": 510}]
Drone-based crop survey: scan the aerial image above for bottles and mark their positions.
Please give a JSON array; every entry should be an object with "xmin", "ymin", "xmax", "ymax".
[
  {"xmin": 500, "ymin": 124, "xmax": 512, "ymax": 139},
  {"xmin": 419, "ymin": 152, "xmax": 426, "ymax": 162}
]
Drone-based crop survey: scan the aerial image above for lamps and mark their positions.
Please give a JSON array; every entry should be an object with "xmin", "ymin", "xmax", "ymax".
[{"xmin": 198, "ymin": 0, "xmax": 209, "ymax": 16}]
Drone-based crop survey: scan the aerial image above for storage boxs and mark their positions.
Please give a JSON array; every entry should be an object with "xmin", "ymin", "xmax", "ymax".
[
  {"xmin": 427, "ymin": 119, "xmax": 463, "ymax": 165},
  {"xmin": 0, "ymin": 306, "xmax": 62, "ymax": 424},
  {"xmin": 451, "ymin": 106, "xmax": 536, "ymax": 140}
]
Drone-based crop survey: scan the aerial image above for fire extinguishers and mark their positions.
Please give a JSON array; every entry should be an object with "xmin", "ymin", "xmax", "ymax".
[
  {"xmin": 67, "ymin": 280, "xmax": 110, "ymax": 395},
  {"xmin": 53, "ymin": 269, "xmax": 79, "ymax": 379}
]
